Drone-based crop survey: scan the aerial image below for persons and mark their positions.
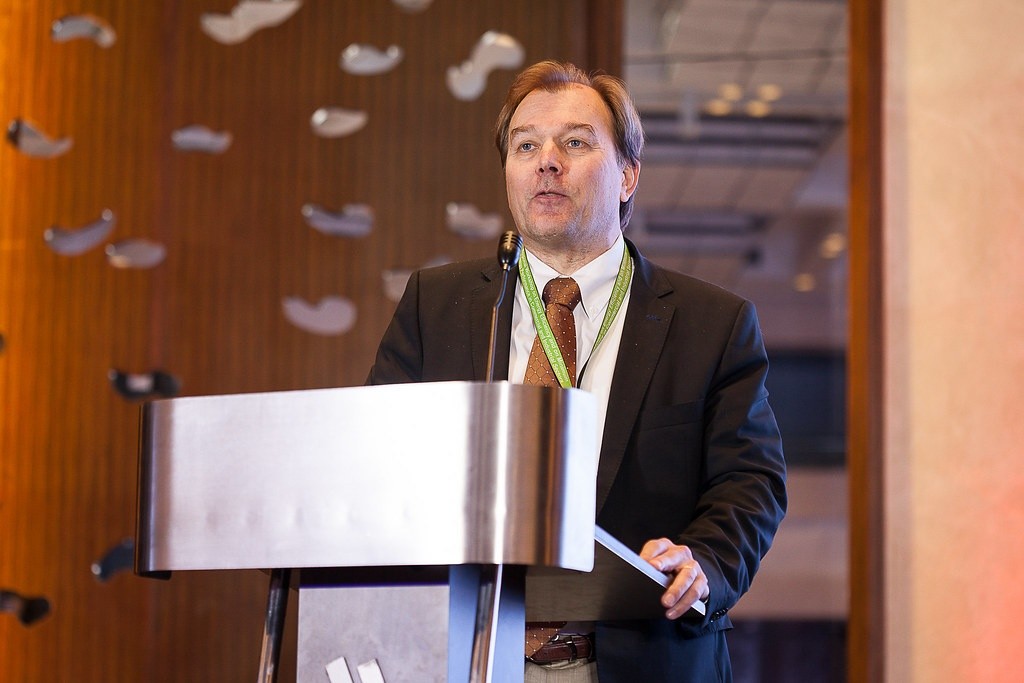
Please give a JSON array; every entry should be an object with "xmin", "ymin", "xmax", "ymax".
[{"xmin": 366, "ymin": 61, "xmax": 788, "ymax": 683}]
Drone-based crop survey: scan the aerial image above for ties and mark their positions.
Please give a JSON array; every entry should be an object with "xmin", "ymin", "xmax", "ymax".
[{"xmin": 525, "ymin": 276, "xmax": 581, "ymax": 665}]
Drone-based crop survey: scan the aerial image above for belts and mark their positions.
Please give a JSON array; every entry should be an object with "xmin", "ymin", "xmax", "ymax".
[{"xmin": 527, "ymin": 632, "xmax": 594, "ymax": 664}]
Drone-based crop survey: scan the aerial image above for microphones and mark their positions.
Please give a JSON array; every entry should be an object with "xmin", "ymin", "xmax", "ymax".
[{"xmin": 487, "ymin": 231, "xmax": 523, "ymax": 381}]
